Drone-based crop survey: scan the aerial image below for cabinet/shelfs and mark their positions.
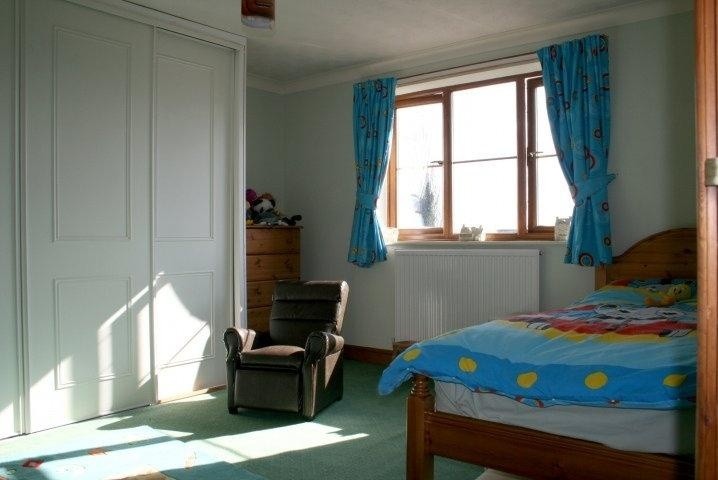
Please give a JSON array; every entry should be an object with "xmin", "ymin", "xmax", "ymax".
[
  {"xmin": 18, "ymin": 0, "xmax": 244, "ymax": 438},
  {"xmin": 0, "ymin": 0, "xmax": 22, "ymax": 445},
  {"xmin": 245, "ymin": 226, "xmax": 303, "ymax": 334}
]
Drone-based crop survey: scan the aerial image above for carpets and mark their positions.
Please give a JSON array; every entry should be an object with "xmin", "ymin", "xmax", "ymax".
[{"xmin": 0, "ymin": 426, "xmax": 270, "ymax": 480}]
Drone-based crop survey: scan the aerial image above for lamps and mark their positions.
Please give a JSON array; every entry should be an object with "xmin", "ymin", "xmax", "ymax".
[{"xmin": 241, "ymin": 0, "xmax": 276, "ymax": 30}]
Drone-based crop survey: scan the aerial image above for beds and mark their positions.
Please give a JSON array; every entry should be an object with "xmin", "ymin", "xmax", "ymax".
[{"xmin": 378, "ymin": 225, "xmax": 697, "ymax": 480}]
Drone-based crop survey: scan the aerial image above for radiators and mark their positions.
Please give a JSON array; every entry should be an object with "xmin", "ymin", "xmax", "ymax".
[{"xmin": 391, "ymin": 250, "xmax": 540, "ymax": 349}]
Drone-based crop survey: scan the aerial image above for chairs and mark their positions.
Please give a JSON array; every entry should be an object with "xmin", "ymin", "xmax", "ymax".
[{"xmin": 223, "ymin": 279, "xmax": 349, "ymax": 420}]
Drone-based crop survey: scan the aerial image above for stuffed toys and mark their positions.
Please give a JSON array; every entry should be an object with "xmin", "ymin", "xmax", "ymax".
[{"xmin": 246, "ymin": 188, "xmax": 302, "ymax": 227}]
